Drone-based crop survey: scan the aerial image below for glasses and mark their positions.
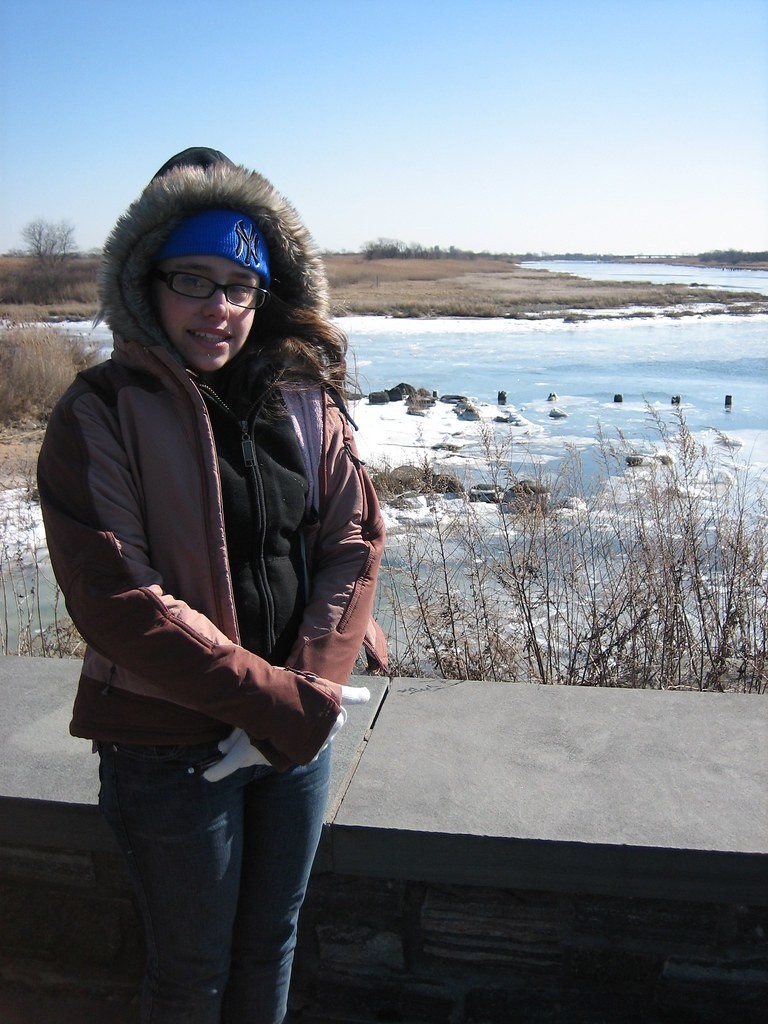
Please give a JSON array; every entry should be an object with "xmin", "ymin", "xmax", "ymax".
[{"xmin": 152, "ymin": 271, "xmax": 270, "ymax": 310}]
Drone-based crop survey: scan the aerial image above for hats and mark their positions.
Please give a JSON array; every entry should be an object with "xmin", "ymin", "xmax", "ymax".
[{"xmin": 155, "ymin": 205, "xmax": 270, "ymax": 287}]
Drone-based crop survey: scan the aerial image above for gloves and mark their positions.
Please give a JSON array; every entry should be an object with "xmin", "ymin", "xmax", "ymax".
[
  {"xmin": 311, "ymin": 685, "xmax": 370, "ymax": 765},
  {"xmin": 202, "ymin": 727, "xmax": 272, "ymax": 783}
]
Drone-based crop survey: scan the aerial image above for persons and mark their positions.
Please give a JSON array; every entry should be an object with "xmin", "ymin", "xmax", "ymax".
[{"xmin": 36, "ymin": 146, "xmax": 387, "ymax": 1024}]
